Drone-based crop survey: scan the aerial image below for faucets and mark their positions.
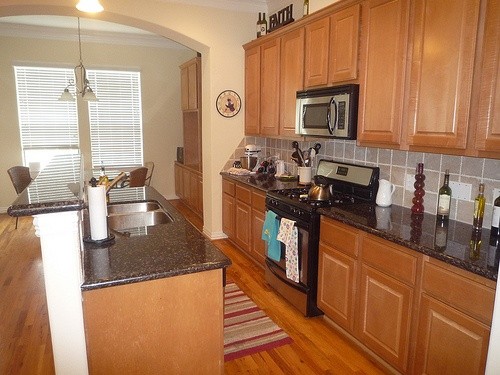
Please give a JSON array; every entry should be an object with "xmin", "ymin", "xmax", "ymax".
[{"xmin": 106, "ymin": 171, "xmax": 131, "ymax": 195}]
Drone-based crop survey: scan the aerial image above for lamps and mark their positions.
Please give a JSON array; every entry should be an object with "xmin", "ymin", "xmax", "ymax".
[{"xmin": 58, "ymin": 16, "xmax": 97, "ymax": 102}]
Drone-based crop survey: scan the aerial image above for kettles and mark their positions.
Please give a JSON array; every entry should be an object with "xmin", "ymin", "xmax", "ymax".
[{"xmin": 308, "ymin": 183, "xmax": 334, "ymax": 201}]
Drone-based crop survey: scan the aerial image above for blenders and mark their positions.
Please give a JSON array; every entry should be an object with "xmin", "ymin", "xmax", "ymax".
[{"xmin": 239, "ymin": 144, "xmax": 261, "ymax": 171}]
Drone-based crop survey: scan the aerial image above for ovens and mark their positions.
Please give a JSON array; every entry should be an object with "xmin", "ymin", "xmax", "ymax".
[{"xmin": 264, "ymin": 205, "xmax": 325, "ymax": 317}]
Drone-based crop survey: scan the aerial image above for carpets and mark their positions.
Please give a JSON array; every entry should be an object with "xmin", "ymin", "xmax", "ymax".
[{"xmin": 224, "ymin": 282, "xmax": 294, "ymax": 361}]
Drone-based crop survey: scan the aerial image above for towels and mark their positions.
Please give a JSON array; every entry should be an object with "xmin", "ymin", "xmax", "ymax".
[
  {"xmin": 260, "ymin": 209, "xmax": 282, "ymax": 262},
  {"xmin": 276, "ymin": 217, "xmax": 299, "ymax": 284}
]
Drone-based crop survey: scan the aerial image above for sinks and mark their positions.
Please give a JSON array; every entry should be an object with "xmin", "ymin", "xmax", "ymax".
[
  {"xmin": 107, "ymin": 212, "xmax": 174, "ymax": 230},
  {"xmin": 107, "ymin": 200, "xmax": 161, "ymax": 213}
]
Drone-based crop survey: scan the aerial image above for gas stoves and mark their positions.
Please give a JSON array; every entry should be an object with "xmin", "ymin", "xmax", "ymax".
[{"xmin": 265, "ymin": 159, "xmax": 380, "ymax": 222}]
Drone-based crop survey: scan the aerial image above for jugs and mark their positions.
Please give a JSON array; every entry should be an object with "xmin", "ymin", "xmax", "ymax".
[
  {"xmin": 376, "ymin": 179, "xmax": 395, "ymax": 206},
  {"xmin": 375, "ymin": 206, "xmax": 393, "ymax": 233}
]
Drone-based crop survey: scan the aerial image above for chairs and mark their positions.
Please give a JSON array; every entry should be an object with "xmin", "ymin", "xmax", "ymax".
[
  {"xmin": 7, "ymin": 166, "xmax": 35, "ymax": 229},
  {"xmin": 124, "ymin": 162, "xmax": 155, "ymax": 187}
]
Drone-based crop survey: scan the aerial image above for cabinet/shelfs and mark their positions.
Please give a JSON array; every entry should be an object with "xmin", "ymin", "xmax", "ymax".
[
  {"xmin": 175, "ymin": 55, "xmax": 203, "ymax": 219},
  {"xmin": 222, "ymin": 175, "xmax": 266, "ymax": 267},
  {"xmin": 317, "ymin": 213, "xmax": 497, "ymax": 375},
  {"xmin": 242, "ymin": 0, "xmax": 500, "ymax": 160}
]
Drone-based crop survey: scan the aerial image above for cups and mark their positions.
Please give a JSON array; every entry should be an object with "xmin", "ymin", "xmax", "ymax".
[{"xmin": 298, "ymin": 167, "xmax": 311, "ymax": 185}]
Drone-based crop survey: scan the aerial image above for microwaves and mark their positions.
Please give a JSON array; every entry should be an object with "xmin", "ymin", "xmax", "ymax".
[{"xmin": 295, "ymin": 84, "xmax": 359, "ymax": 140}]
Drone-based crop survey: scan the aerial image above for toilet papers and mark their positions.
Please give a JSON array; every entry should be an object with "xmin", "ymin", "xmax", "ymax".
[
  {"xmin": 87, "ymin": 185, "xmax": 108, "ymax": 240},
  {"xmin": 298, "ymin": 167, "xmax": 312, "ymax": 184}
]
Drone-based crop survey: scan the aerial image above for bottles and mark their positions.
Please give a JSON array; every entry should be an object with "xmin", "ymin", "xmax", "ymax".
[
  {"xmin": 487, "ymin": 238, "xmax": 500, "ymax": 271},
  {"xmin": 473, "ymin": 184, "xmax": 486, "ymax": 230},
  {"xmin": 434, "ymin": 220, "xmax": 449, "ymax": 252},
  {"xmin": 437, "ymin": 170, "xmax": 452, "ymax": 222},
  {"xmin": 491, "ymin": 196, "xmax": 500, "ymax": 237},
  {"xmin": 303, "ymin": 0, "xmax": 309, "ymax": 17},
  {"xmin": 470, "ymin": 231, "xmax": 481, "ymax": 260},
  {"xmin": 261, "ymin": 13, "xmax": 267, "ymax": 36},
  {"xmin": 257, "ymin": 12, "xmax": 261, "ymax": 38}
]
